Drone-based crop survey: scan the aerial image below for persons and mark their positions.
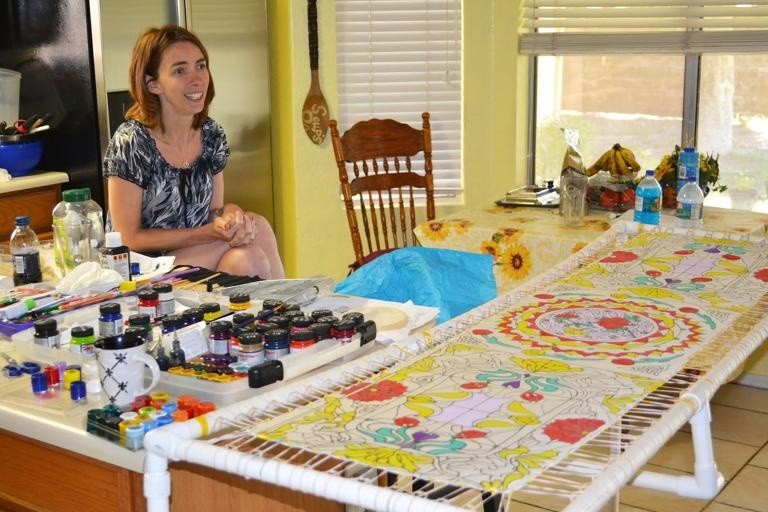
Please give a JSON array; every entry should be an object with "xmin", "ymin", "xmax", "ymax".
[{"xmin": 102, "ymin": 25, "xmax": 287, "ymax": 280}]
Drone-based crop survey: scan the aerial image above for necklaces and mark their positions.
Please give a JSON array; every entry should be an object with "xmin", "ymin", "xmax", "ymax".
[{"xmin": 156, "ymin": 125, "xmax": 200, "ymax": 168}]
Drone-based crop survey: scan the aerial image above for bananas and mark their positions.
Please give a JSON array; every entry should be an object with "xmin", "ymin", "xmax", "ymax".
[{"xmin": 586, "ymin": 143, "xmax": 640, "ymax": 182}]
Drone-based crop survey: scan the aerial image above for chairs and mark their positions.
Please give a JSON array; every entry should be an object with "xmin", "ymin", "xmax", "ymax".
[{"xmin": 332, "ymin": 113, "xmax": 435, "ymax": 270}]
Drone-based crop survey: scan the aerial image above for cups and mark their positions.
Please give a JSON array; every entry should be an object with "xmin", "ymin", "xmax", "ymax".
[
  {"xmin": 93, "ymin": 335, "xmax": 160, "ymax": 406},
  {"xmin": 52, "ymin": 218, "xmax": 92, "ymax": 273},
  {"xmin": 562, "ymin": 175, "xmax": 588, "ymax": 229}
]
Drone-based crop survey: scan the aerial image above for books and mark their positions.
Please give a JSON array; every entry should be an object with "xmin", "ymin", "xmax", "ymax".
[
  {"xmin": 506, "ymin": 185, "xmax": 557, "ymax": 200},
  {"xmin": 495, "ymin": 194, "xmax": 560, "ymax": 209}
]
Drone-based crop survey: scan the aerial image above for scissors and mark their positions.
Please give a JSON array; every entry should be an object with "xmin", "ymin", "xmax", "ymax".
[{"xmin": 1, "ymin": 350, "xmax": 42, "ymax": 378}]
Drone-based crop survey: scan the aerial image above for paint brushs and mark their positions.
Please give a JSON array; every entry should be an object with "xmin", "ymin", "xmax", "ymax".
[
  {"xmin": 0, "ymin": 284, "xmax": 47, "ymax": 310},
  {"xmin": 58, "ymin": 291, "xmax": 122, "ymax": 311},
  {"xmin": 231, "ymin": 302, "xmax": 286, "ymax": 334},
  {"xmin": 151, "ymin": 267, "xmax": 228, "ymax": 297}
]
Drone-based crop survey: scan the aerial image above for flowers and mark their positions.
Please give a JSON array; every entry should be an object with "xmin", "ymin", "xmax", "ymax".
[
  {"xmin": 480, "ymin": 226, "xmax": 531, "ymax": 281},
  {"xmin": 423, "ymin": 218, "xmax": 472, "ymax": 243}
]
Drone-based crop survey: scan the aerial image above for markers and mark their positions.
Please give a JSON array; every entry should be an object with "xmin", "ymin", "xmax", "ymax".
[{"xmin": 13, "ymin": 114, "xmax": 54, "ymax": 139}]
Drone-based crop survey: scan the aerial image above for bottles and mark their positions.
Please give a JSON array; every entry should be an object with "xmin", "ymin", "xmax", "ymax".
[
  {"xmin": 673, "ymin": 176, "xmax": 703, "ymax": 229},
  {"xmin": 632, "ymin": 169, "xmax": 661, "ymax": 226},
  {"xmin": 8, "ymin": 216, "xmax": 42, "ymax": 287},
  {"xmin": 71, "ymin": 326, "xmax": 95, "ymax": 356},
  {"xmin": 101, "ymin": 231, "xmax": 131, "ymax": 283},
  {"xmin": 676, "ymin": 147, "xmax": 699, "ymax": 191},
  {"xmin": 53, "ymin": 187, "xmax": 105, "ymax": 279},
  {"xmin": 33, "ymin": 319, "xmax": 60, "ymax": 350},
  {"xmin": 98, "ymin": 282, "xmax": 364, "ymax": 367}
]
